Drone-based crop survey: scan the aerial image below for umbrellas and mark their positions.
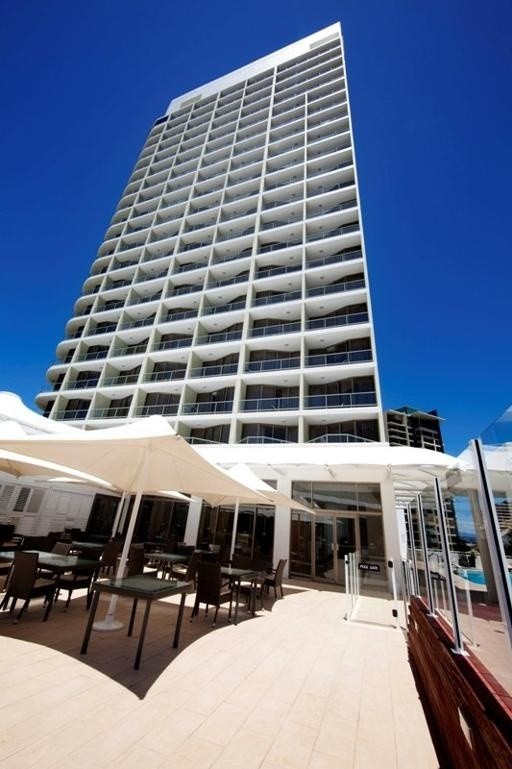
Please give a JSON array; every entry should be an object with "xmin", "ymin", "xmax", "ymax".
[
  {"xmin": 186, "ymin": 436, "xmax": 323, "ymax": 578},
  {"xmin": 0, "ymin": 400, "xmax": 275, "ymax": 624},
  {"xmin": 0, "ymin": 390, "xmax": 95, "ymax": 485}
]
